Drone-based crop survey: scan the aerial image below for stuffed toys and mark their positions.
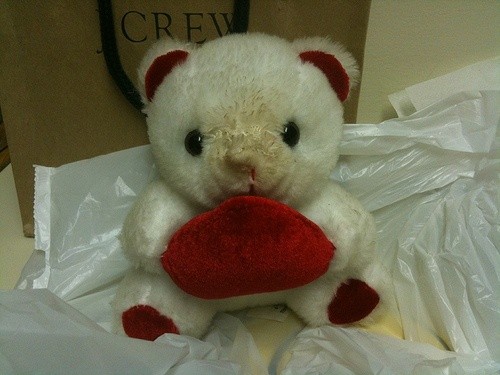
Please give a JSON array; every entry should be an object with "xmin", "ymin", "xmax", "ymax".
[{"xmin": 120, "ymin": 35, "xmax": 386, "ymax": 343}]
